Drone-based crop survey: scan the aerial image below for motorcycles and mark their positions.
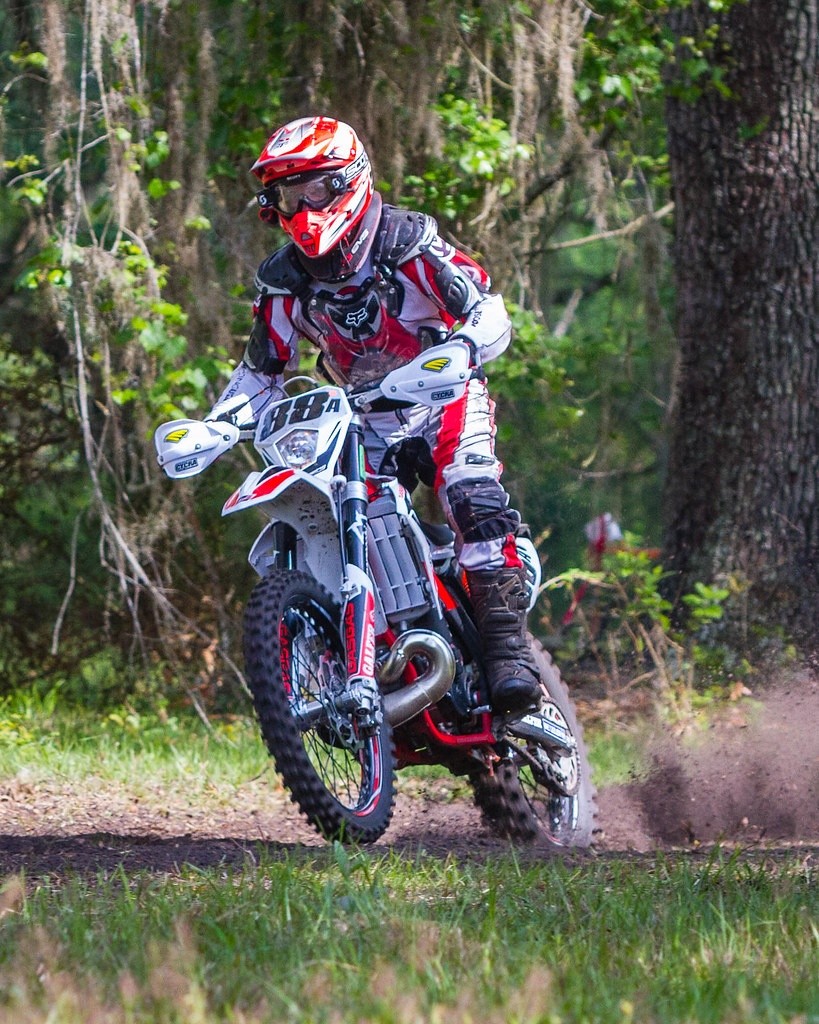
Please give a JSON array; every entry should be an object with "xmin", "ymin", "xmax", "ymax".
[{"xmin": 153, "ymin": 341, "xmax": 605, "ymax": 856}]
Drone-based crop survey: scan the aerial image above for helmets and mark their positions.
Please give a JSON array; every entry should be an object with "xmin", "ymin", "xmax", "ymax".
[{"xmin": 249, "ymin": 116, "xmax": 373, "ymax": 259}]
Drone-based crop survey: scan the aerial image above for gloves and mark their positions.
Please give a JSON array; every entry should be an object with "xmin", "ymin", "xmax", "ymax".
[{"xmin": 449, "ymin": 335, "xmax": 477, "ymax": 368}]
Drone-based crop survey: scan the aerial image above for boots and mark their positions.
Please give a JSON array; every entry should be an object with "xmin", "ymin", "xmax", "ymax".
[
  {"xmin": 465, "ymin": 567, "xmax": 543, "ymax": 714},
  {"xmin": 316, "ymin": 645, "xmax": 355, "ymax": 750}
]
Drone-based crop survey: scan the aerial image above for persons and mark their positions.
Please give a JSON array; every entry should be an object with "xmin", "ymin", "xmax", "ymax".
[
  {"xmin": 581, "ymin": 513, "xmax": 661, "ymax": 656},
  {"xmin": 202, "ymin": 116, "xmax": 542, "ymax": 713}
]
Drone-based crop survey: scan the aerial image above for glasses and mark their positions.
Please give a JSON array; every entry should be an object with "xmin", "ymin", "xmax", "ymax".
[{"xmin": 255, "ymin": 169, "xmax": 347, "ymax": 218}]
